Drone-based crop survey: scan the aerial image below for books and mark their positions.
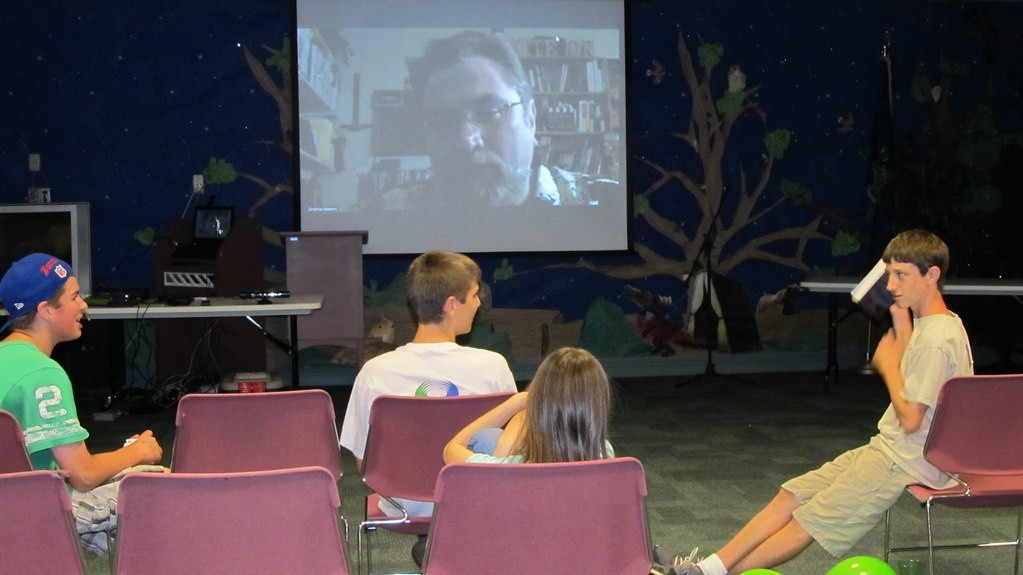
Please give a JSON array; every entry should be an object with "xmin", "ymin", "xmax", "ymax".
[
  {"xmin": 298, "ymin": 28, "xmax": 337, "ymax": 108},
  {"xmin": 521, "ymin": 56, "xmax": 621, "ymax": 92},
  {"xmin": 532, "ymin": 134, "xmax": 619, "ymax": 182},
  {"xmin": 300, "ymin": 116, "xmax": 336, "ymax": 168},
  {"xmin": 576, "ymin": 96, "xmax": 622, "ymax": 133}
]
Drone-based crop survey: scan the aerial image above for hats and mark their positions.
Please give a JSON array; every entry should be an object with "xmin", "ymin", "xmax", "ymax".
[{"xmin": 0, "ymin": 253, "xmax": 73, "ymax": 336}]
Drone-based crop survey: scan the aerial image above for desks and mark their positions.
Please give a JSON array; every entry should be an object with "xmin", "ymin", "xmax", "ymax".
[
  {"xmin": 800, "ymin": 282, "xmax": 1023, "ymax": 393},
  {"xmin": 0, "ymin": 293, "xmax": 325, "ymax": 391},
  {"xmin": 0, "ymin": 202, "xmax": 91, "ymax": 298}
]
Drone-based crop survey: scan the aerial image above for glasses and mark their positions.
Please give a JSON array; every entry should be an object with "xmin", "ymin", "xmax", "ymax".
[{"xmin": 423, "ymin": 99, "xmax": 526, "ymax": 137}]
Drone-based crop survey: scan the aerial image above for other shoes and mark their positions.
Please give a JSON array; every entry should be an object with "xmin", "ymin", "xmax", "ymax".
[{"xmin": 412, "ymin": 534, "xmax": 428, "ymax": 568}]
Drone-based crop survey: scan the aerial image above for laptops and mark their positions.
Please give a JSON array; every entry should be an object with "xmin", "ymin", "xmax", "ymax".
[{"xmin": 169, "ymin": 205, "xmax": 236, "ymax": 261}]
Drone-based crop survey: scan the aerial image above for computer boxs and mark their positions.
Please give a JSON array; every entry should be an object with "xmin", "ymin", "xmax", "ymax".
[{"xmin": 70, "ymin": 316, "xmax": 128, "ymax": 394}]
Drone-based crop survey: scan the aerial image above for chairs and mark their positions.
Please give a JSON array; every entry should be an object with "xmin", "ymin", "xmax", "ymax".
[
  {"xmin": 0, "ymin": 470, "xmax": 89, "ymax": 575},
  {"xmin": 112, "ymin": 466, "xmax": 351, "ymax": 575},
  {"xmin": 883, "ymin": 374, "xmax": 1023, "ymax": 575},
  {"xmin": 422, "ymin": 457, "xmax": 654, "ymax": 575},
  {"xmin": 171, "ymin": 388, "xmax": 349, "ymax": 543},
  {"xmin": 357, "ymin": 392, "xmax": 514, "ymax": 575}
]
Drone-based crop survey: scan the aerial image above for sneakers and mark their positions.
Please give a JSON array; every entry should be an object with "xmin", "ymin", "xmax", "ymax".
[{"xmin": 649, "ymin": 543, "xmax": 706, "ymax": 575}]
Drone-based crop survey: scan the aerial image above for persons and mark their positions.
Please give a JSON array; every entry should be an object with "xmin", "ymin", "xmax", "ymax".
[
  {"xmin": 0, "ymin": 253, "xmax": 171, "ymax": 556},
  {"xmin": 366, "ymin": 30, "xmax": 625, "ymax": 206},
  {"xmin": 443, "ymin": 346, "xmax": 614, "ymax": 463},
  {"xmin": 650, "ymin": 228, "xmax": 974, "ymax": 575},
  {"xmin": 338, "ymin": 250, "xmax": 519, "ymax": 568}
]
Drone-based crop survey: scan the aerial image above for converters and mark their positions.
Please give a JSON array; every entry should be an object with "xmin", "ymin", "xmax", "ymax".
[{"xmin": 94, "ymin": 412, "xmax": 118, "ymax": 421}]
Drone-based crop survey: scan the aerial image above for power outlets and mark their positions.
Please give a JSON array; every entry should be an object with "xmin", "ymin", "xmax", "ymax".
[{"xmin": 39, "ymin": 187, "xmax": 50, "ymax": 203}]
